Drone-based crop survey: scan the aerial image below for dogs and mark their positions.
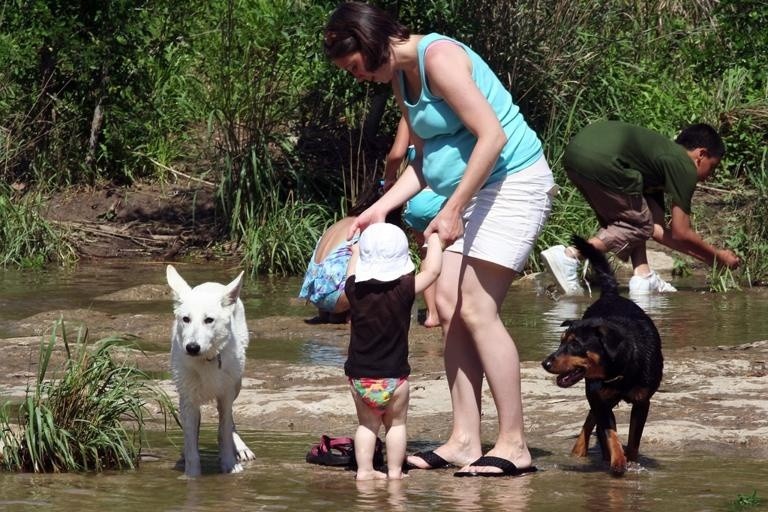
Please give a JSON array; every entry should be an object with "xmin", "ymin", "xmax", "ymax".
[
  {"xmin": 166, "ymin": 264, "xmax": 257, "ymax": 479},
  {"xmin": 542, "ymin": 234, "xmax": 664, "ymax": 477}
]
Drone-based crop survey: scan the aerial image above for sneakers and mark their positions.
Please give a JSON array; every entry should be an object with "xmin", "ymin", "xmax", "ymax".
[
  {"xmin": 539, "ymin": 245, "xmax": 586, "ymax": 296},
  {"xmin": 628, "ymin": 270, "xmax": 679, "ymax": 294}
]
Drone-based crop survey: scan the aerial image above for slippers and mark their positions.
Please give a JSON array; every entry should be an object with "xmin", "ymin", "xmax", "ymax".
[
  {"xmin": 453, "ymin": 455, "xmax": 538, "ymax": 477},
  {"xmin": 403, "ymin": 449, "xmax": 460, "ymax": 471}
]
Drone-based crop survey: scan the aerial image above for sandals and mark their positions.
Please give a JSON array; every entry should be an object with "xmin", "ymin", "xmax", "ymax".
[
  {"xmin": 306, "ymin": 435, "xmax": 354, "ymax": 466},
  {"xmin": 372, "ymin": 437, "xmax": 384, "ymax": 469}
]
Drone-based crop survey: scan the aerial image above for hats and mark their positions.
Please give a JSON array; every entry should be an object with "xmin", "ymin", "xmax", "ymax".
[{"xmin": 354, "ymin": 222, "xmax": 415, "ymax": 284}]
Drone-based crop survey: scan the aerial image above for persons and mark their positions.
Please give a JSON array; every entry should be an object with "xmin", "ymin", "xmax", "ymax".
[
  {"xmin": 343, "ymin": 225, "xmax": 448, "ymax": 484},
  {"xmin": 402, "ymin": 135, "xmax": 453, "ymax": 326},
  {"xmin": 321, "ymin": 2, "xmax": 563, "ymax": 478},
  {"xmin": 297, "ymin": 183, "xmax": 391, "ymax": 325},
  {"xmin": 538, "ymin": 114, "xmax": 742, "ymax": 301}
]
